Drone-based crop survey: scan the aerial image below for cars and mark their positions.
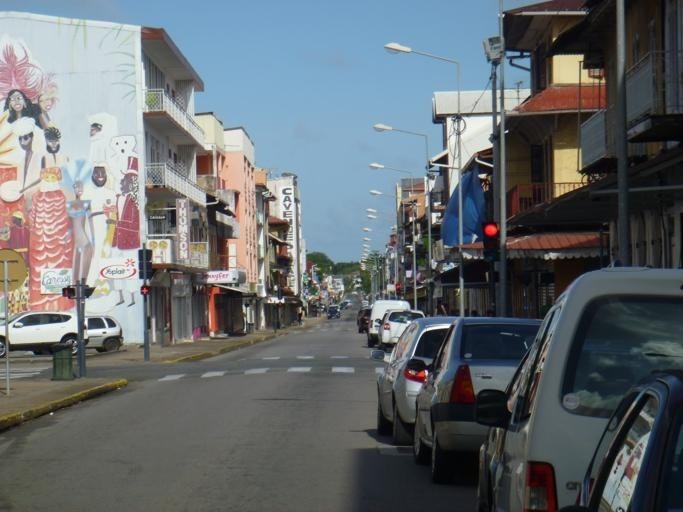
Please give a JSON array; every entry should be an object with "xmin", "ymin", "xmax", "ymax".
[
  {"xmin": 413, "ymin": 316, "xmax": 543, "ymax": 482},
  {"xmin": 376, "ymin": 315, "xmax": 458, "ymax": 445},
  {"xmin": 339, "ymin": 300, "xmax": 352, "ymax": 310},
  {"xmin": 358, "ymin": 300, "xmax": 426, "ymax": 351}
]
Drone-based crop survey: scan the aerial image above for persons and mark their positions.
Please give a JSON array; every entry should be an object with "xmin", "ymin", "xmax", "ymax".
[
  {"xmin": 10, "ymin": 117, "xmax": 48, "ymax": 217},
  {"xmin": 17, "ymin": 123, "xmax": 142, "ymax": 310},
  {"xmin": 0, "ymin": 88, "xmax": 44, "ymax": 211}
]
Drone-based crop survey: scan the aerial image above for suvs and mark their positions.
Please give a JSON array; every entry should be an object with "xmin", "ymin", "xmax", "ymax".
[
  {"xmin": 313, "ymin": 303, "xmax": 326, "ymax": 312},
  {"xmin": 0, "ymin": 311, "xmax": 124, "ymax": 359},
  {"xmin": 328, "ymin": 304, "xmax": 340, "ymax": 319}
]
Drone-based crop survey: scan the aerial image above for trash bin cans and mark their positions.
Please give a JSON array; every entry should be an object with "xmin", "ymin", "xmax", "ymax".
[{"xmin": 51, "ymin": 343, "xmax": 73, "ymax": 380}]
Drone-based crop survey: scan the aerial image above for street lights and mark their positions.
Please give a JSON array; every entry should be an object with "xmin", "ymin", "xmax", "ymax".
[
  {"xmin": 383, "ymin": 42, "xmax": 466, "ymax": 317},
  {"xmin": 360, "ymin": 122, "xmax": 433, "ymax": 309}
]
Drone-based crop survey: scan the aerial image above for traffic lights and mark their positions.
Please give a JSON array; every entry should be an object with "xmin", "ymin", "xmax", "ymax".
[
  {"xmin": 484, "ymin": 222, "xmax": 499, "ymax": 263},
  {"xmin": 62, "ymin": 288, "xmax": 75, "ymax": 297},
  {"xmin": 141, "ymin": 285, "xmax": 151, "ymax": 295},
  {"xmin": 84, "ymin": 287, "xmax": 96, "ymax": 297}
]
